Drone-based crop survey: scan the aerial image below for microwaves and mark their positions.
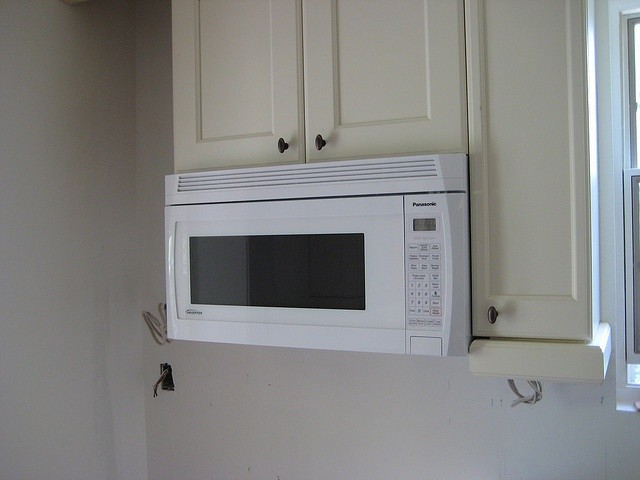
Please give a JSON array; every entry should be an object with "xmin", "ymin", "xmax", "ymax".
[{"xmin": 165, "ymin": 155, "xmax": 470, "ymax": 356}]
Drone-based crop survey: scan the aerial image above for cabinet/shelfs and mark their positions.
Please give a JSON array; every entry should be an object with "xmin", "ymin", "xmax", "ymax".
[
  {"xmin": 170, "ymin": 0, "xmax": 468, "ymax": 174},
  {"xmin": 464, "ymin": 0, "xmax": 614, "ymax": 385}
]
